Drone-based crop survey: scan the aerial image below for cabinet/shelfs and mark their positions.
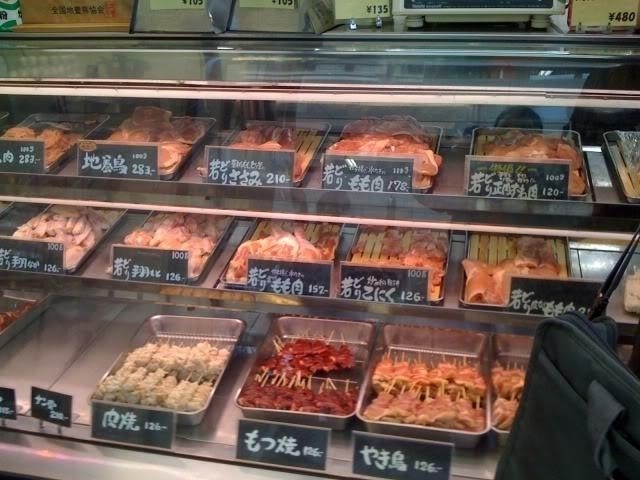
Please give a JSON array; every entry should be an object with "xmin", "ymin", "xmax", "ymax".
[{"xmin": 0, "ymin": 31, "xmax": 640, "ymax": 480}]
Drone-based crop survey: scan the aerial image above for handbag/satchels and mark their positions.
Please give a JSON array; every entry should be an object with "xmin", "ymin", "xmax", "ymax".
[{"xmin": 494, "ymin": 311, "xmax": 640, "ymax": 480}]
